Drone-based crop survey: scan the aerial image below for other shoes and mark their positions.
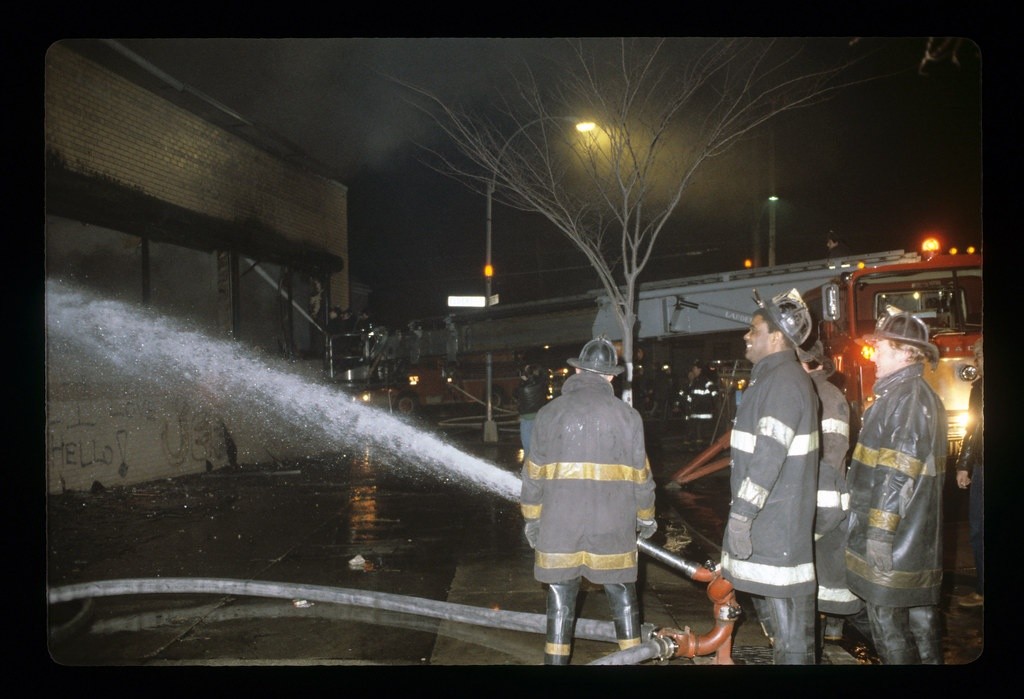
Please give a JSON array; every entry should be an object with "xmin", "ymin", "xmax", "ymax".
[{"xmin": 956, "ymin": 591, "xmax": 984, "ymax": 607}]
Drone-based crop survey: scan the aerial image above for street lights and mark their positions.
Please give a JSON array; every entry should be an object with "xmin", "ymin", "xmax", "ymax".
[
  {"xmin": 481, "ymin": 117, "xmax": 599, "ymax": 522},
  {"xmin": 752, "ymin": 195, "xmax": 779, "ymax": 269}
]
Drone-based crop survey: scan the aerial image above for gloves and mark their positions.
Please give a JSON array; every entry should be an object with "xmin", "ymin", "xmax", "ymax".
[
  {"xmin": 634, "ymin": 516, "xmax": 658, "ymax": 539},
  {"xmin": 865, "ymin": 539, "xmax": 894, "ymax": 574},
  {"xmin": 727, "ymin": 514, "xmax": 753, "ymax": 560},
  {"xmin": 525, "ymin": 521, "xmax": 542, "ymax": 548}
]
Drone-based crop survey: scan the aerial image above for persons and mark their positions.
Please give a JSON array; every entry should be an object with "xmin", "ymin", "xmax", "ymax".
[
  {"xmin": 320, "ymin": 307, "xmax": 375, "ymax": 369},
  {"xmin": 720, "ymin": 299, "xmax": 866, "ymax": 665},
  {"xmin": 845, "ymin": 313, "xmax": 948, "ymax": 665},
  {"xmin": 955, "ymin": 336, "xmax": 984, "ymax": 607},
  {"xmin": 633, "ymin": 341, "xmax": 721, "ymax": 453},
  {"xmin": 519, "ymin": 336, "xmax": 658, "ymax": 665},
  {"xmin": 826, "ymin": 230, "xmax": 848, "ymax": 258}
]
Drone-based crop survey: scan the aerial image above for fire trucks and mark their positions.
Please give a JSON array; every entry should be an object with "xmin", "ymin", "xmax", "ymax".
[{"xmin": 322, "ymin": 232, "xmax": 984, "ymax": 488}]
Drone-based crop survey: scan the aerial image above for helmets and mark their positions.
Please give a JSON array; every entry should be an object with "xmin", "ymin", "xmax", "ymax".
[
  {"xmin": 566, "ymin": 334, "xmax": 625, "ymax": 377},
  {"xmin": 796, "ymin": 339, "xmax": 836, "ymax": 378},
  {"xmin": 519, "ymin": 364, "xmax": 538, "ymax": 383},
  {"xmin": 752, "ymin": 289, "xmax": 815, "ymax": 363},
  {"xmin": 864, "ymin": 310, "xmax": 939, "ymax": 372}
]
